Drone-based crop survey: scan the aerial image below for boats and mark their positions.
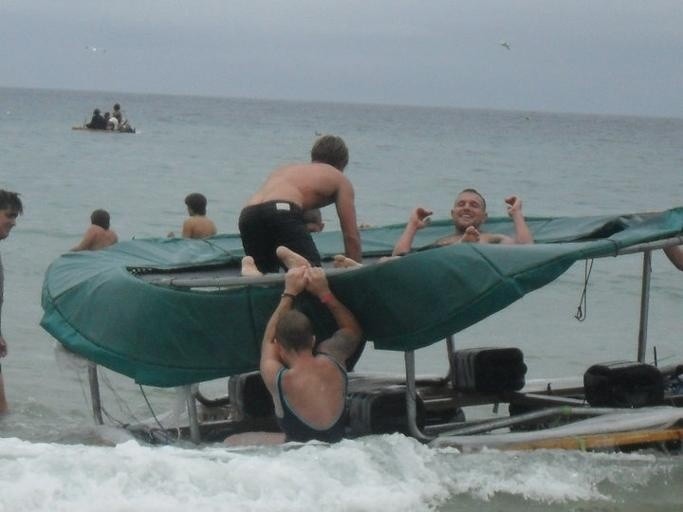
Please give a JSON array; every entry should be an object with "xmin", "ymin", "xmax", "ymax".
[
  {"xmin": 40, "ymin": 207, "xmax": 683, "ymax": 456},
  {"xmin": 73, "ymin": 121, "xmax": 136, "ymax": 134}
]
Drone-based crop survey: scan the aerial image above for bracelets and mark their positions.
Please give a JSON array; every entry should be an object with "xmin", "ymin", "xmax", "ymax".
[
  {"xmin": 319, "ymin": 291, "xmax": 333, "ymax": 304},
  {"xmin": 280, "ymin": 292, "xmax": 297, "ymax": 303}
]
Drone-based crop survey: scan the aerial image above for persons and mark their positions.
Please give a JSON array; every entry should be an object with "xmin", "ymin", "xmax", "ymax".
[
  {"xmin": 662, "ymin": 242, "xmax": 682, "ymax": 272},
  {"xmin": 86, "ymin": 103, "xmax": 132, "ymax": 132},
  {"xmin": 330, "ymin": 188, "xmax": 535, "ymax": 269},
  {"xmin": 237, "ymin": 134, "xmax": 363, "ymax": 279},
  {"xmin": 218, "ymin": 264, "xmax": 365, "ymax": 450},
  {"xmin": 69, "ymin": 208, "xmax": 118, "ymax": 251},
  {"xmin": 0, "ymin": 188, "xmax": 24, "ymax": 420},
  {"xmin": 165, "ymin": 192, "xmax": 218, "ymax": 240}
]
